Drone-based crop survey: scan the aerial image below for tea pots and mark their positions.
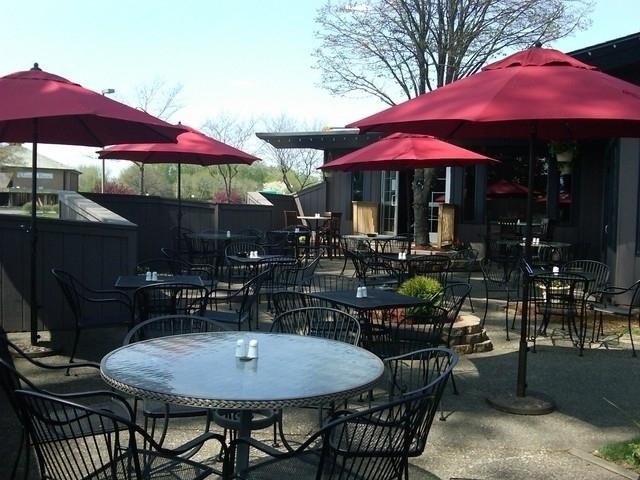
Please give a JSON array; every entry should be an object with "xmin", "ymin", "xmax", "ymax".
[{"xmin": 237, "ymin": 251, "xmax": 250, "ymax": 258}]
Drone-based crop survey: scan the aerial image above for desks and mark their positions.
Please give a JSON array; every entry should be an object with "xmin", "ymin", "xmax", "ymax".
[
  {"xmin": 114, "ymin": 275, "xmax": 206, "ymax": 288},
  {"xmin": 379, "ymin": 253, "xmax": 449, "ymax": 279},
  {"xmin": 338, "ymin": 233, "xmax": 408, "ymax": 279},
  {"xmin": 100, "ymin": 330, "xmax": 385, "ymax": 480},
  {"xmin": 297, "ymin": 215, "xmax": 331, "ymax": 259},
  {"xmin": 497, "ymin": 221, "xmax": 543, "ymax": 234},
  {"xmin": 519, "ymin": 240, "xmax": 571, "ymax": 258},
  {"xmin": 529, "ymin": 271, "xmax": 596, "ymax": 343},
  {"xmin": 186, "ymin": 232, "xmax": 246, "ymax": 283},
  {"xmin": 270, "ymin": 230, "xmax": 310, "ymax": 254},
  {"xmin": 305, "ymin": 287, "xmax": 433, "ymax": 402},
  {"xmin": 226, "ymin": 254, "xmax": 296, "ymax": 264}
]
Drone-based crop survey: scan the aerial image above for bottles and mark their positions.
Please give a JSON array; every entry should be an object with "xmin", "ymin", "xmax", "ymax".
[
  {"xmin": 362, "ymin": 286, "xmax": 368, "ymax": 297},
  {"xmin": 145, "ymin": 271, "xmax": 152, "ymax": 281},
  {"xmin": 533, "ymin": 238, "xmax": 536, "ymax": 245},
  {"xmin": 249, "ymin": 250, "xmax": 254, "ymax": 259},
  {"xmin": 151, "ymin": 271, "xmax": 158, "ymax": 281},
  {"xmin": 226, "ymin": 229, "xmax": 231, "ymax": 237},
  {"xmin": 254, "ymin": 250, "xmax": 258, "ymax": 258},
  {"xmin": 537, "ymin": 238, "xmax": 540, "ymax": 245},
  {"xmin": 402, "ymin": 252, "xmax": 407, "ymax": 259}
]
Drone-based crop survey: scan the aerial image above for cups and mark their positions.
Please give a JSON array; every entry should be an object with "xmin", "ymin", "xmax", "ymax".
[
  {"xmin": 254, "ymin": 251, "xmax": 258, "ymax": 258},
  {"xmin": 152, "ymin": 272, "xmax": 158, "ymax": 281},
  {"xmin": 361, "ymin": 287, "xmax": 367, "ymax": 297},
  {"xmin": 315, "ymin": 213, "xmax": 320, "ymax": 217},
  {"xmin": 250, "ymin": 251, "xmax": 254, "ymax": 258},
  {"xmin": 227, "ymin": 231, "xmax": 230, "ymax": 237},
  {"xmin": 403, "ymin": 253, "xmax": 407, "ymax": 259},
  {"xmin": 295, "ymin": 228, "xmax": 299, "ymax": 231},
  {"xmin": 145, "ymin": 272, "xmax": 151, "ymax": 281}
]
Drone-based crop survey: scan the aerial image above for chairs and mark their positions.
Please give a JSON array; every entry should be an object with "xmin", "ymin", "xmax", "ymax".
[
  {"xmin": 480, "ymin": 256, "xmax": 533, "ymax": 341},
  {"xmin": 269, "ymin": 306, "xmax": 361, "ymax": 448},
  {"xmin": 390, "ymin": 282, "xmax": 472, "ymax": 422},
  {"xmin": 485, "ymin": 215, "xmax": 559, "ymax": 271},
  {"xmin": 530, "ymin": 272, "xmax": 589, "ymax": 357},
  {"xmin": 446, "ymin": 246, "xmax": 479, "ymax": 313},
  {"xmin": 298, "ymin": 273, "xmax": 362, "ymax": 306},
  {"xmin": 271, "ymin": 291, "xmax": 350, "ymax": 333},
  {"xmin": 188, "ymin": 268, "xmax": 271, "ymax": 331},
  {"xmin": 559, "ymin": 259, "xmax": 610, "ymax": 344},
  {"xmin": 133, "ymin": 282, "xmax": 209, "ymax": 323},
  {"xmin": 0, "ymin": 324, "xmax": 229, "ymax": 480},
  {"xmin": 269, "ymin": 210, "xmax": 344, "ymax": 261},
  {"xmin": 225, "ymin": 241, "xmax": 326, "ymax": 310},
  {"xmin": 50, "ymin": 267, "xmax": 134, "ymax": 377},
  {"xmin": 122, "ymin": 314, "xmax": 235, "ymax": 467},
  {"xmin": 579, "ymin": 280, "xmax": 640, "ymax": 358},
  {"xmin": 343, "ymin": 234, "xmax": 452, "ymax": 286},
  {"xmin": 160, "ymin": 225, "xmax": 264, "ymax": 283},
  {"xmin": 222, "ymin": 346, "xmax": 460, "ymax": 480}
]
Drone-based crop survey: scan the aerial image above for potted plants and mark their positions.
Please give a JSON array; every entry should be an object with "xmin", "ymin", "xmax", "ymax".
[{"xmin": 539, "ymin": 280, "xmax": 570, "ymax": 303}]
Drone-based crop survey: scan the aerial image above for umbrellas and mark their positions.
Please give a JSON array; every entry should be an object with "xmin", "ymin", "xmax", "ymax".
[
  {"xmin": 538, "ymin": 192, "xmax": 573, "ymax": 212},
  {"xmin": 318, "ymin": 132, "xmax": 499, "ymax": 257},
  {"xmin": 434, "ymin": 195, "xmax": 446, "ymax": 203},
  {"xmin": 96, "ymin": 121, "xmax": 261, "ymax": 239},
  {"xmin": 1, "ymin": 63, "xmax": 188, "ymax": 345},
  {"xmin": 345, "ymin": 47, "xmax": 639, "ymax": 395},
  {"xmin": 484, "ymin": 179, "xmax": 530, "ymax": 217}
]
